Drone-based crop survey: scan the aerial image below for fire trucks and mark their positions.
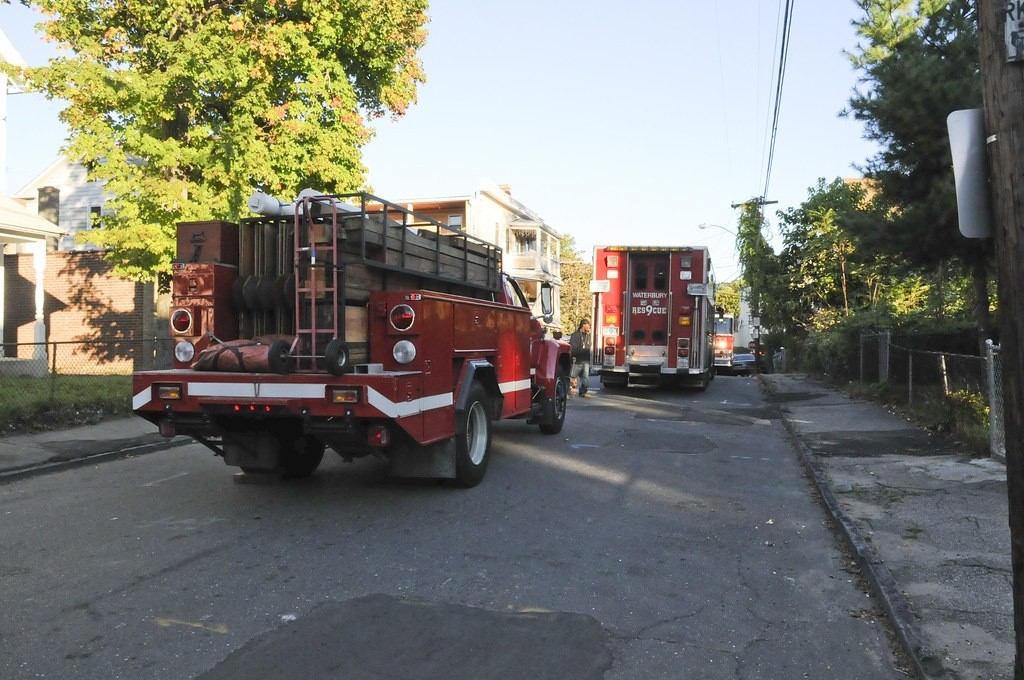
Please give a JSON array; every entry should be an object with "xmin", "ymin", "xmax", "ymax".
[
  {"xmin": 130, "ymin": 187, "xmax": 574, "ymax": 491},
  {"xmin": 714, "ymin": 314, "xmax": 734, "ymax": 375},
  {"xmin": 588, "ymin": 245, "xmax": 724, "ymax": 393}
]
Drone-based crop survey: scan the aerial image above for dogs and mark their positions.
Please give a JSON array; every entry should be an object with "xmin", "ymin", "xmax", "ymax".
[{"xmin": 566, "ymin": 377, "xmax": 577, "ymax": 399}]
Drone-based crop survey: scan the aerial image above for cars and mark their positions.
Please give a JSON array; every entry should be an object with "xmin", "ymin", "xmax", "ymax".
[{"xmin": 733, "ymin": 354, "xmax": 755, "ymax": 376}]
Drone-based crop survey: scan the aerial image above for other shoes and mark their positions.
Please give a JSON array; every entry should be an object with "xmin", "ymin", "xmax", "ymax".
[{"xmin": 579, "ymin": 393, "xmax": 590, "ymax": 397}]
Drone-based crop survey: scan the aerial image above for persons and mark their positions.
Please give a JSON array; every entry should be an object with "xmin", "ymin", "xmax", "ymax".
[{"xmin": 567, "ymin": 319, "xmax": 591, "ymax": 398}]
